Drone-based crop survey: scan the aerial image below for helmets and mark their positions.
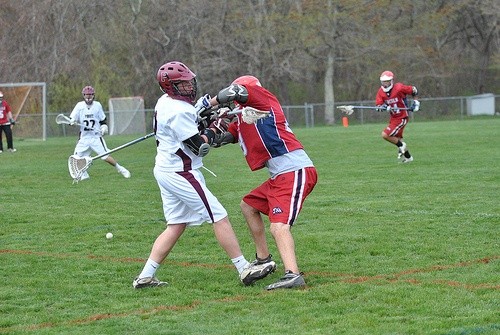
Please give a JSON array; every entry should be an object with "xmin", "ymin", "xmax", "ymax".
[
  {"xmin": 380, "ymin": 71, "xmax": 394, "ymax": 92},
  {"xmin": 82, "ymin": 86, "xmax": 95, "ymax": 105},
  {"xmin": 157, "ymin": 62, "xmax": 196, "ymax": 102},
  {"xmin": 230, "ymin": 75, "xmax": 261, "ymax": 107}
]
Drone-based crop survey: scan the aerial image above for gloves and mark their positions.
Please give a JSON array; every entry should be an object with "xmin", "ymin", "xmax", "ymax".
[
  {"xmin": 70, "ymin": 119, "xmax": 77, "ymax": 127},
  {"xmin": 411, "ymin": 100, "xmax": 420, "ymax": 112},
  {"xmin": 100, "ymin": 124, "xmax": 108, "ymax": 135},
  {"xmin": 376, "ymin": 105, "xmax": 387, "ymax": 112},
  {"xmin": 195, "ymin": 93, "xmax": 235, "ymax": 134}
]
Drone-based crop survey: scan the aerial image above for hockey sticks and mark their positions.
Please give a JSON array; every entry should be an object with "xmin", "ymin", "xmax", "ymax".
[
  {"xmin": 336, "ymin": 106, "xmax": 421, "ymax": 115},
  {"xmin": 226, "ymin": 107, "xmax": 271, "ymax": 125},
  {"xmin": 55, "ymin": 113, "xmax": 102, "ymax": 132},
  {"xmin": 67, "ymin": 132, "xmax": 155, "ymax": 180}
]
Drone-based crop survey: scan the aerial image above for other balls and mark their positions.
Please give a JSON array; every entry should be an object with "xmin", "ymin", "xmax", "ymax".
[{"xmin": 106, "ymin": 232, "xmax": 114, "ymax": 240}]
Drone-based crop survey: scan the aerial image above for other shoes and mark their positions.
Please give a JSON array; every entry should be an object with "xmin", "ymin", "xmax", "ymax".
[
  {"xmin": 7, "ymin": 148, "xmax": 16, "ymax": 152},
  {"xmin": 397, "ymin": 142, "xmax": 406, "ymax": 159},
  {"xmin": 117, "ymin": 166, "xmax": 131, "ymax": 178},
  {"xmin": 399, "ymin": 155, "xmax": 414, "ymax": 163},
  {"xmin": 81, "ymin": 171, "xmax": 90, "ymax": 181}
]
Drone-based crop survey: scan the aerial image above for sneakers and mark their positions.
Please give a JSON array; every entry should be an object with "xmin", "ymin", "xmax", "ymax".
[
  {"xmin": 133, "ymin": 274, "xmax": 168, "ymax": 289},
  {"xmin": 240, "ymin": 261, "xmax": 276, "ymax": 286},
  {"xmin": 250, "ymin": 253, "xmax": 273, "ymax": 267},
  {"xmin": 266, "ymin": 270, "xmax": 305, "ymax": 290}
]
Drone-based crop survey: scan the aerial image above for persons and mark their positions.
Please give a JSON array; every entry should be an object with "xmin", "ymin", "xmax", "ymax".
[
  {"xmin": 194, "ymin": 75, "xmax": 318, "ymax": 288},
  {"xmin": 376, "ymin": 71, "xmax": 419, "ymax": 164},
  {"xmin": 0, "ymin": 91, "xmax": 16, "ymax": 152},
  {"xmin": 131, "ymin": 61, "xmax": 275, "ymax": 290},
  {"xmin": 67, "ymin": 86, "xmax": 130, "ymax": 179}
]
